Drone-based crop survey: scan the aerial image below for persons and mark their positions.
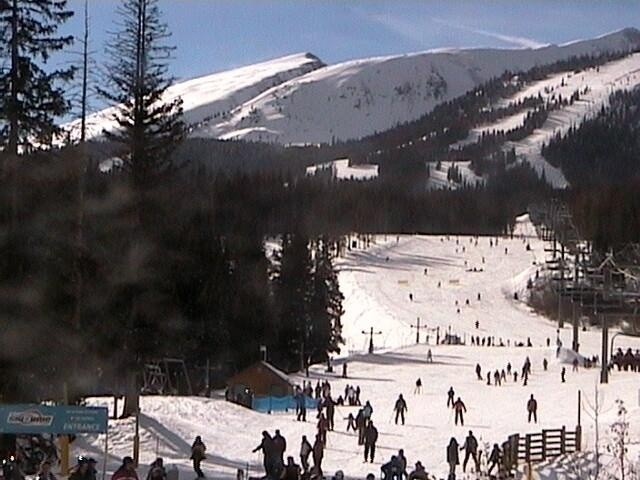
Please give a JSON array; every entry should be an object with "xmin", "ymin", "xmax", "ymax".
[
  {"xmin": 384, "ymin": 227, "xmax": 640, "ymax": 426},
  {"xmin": 0, "ymin": 373, "xmax": 484, "ymax": 480},
  {"xmin": 341, "ymin": 361, "xmax": 348, "ymax": 379},
  {"xmin": 329, "ymin": 356, "xmax": 334, "ymax": 373},
  {"xmin": 486, "ymin": 440, "xmax": 504, "ymax": 479}
]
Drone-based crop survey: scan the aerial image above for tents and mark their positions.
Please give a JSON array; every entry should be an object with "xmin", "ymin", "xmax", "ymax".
[{"xmin": 227, "ymin": 361, "xmax": 322, "ymax": 413}]
